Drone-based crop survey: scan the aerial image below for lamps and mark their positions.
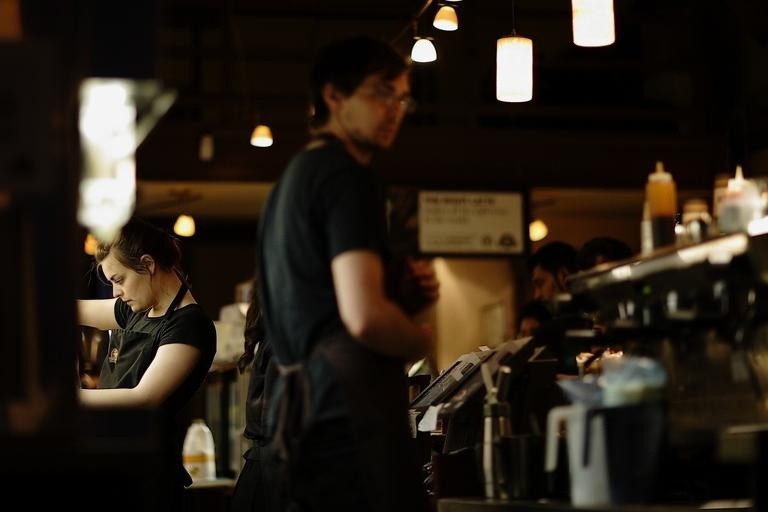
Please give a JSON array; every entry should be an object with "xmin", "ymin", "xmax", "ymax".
[
  {"xmin": 249, "ymin": 123, "xmax": 274, "ymax": 147},
  {"xmin": 395, "ymin": 0, "xmax": 464, "ymax": 63},
  {"xmin": 495, "ymin": 35, "xmax": 535, "ymax": 103},
  {"xmin": 571, "ymin": 0, "xmax": 616, "ymax": 48},
  {"xmin": 173, "ymin": 208, "xmax": 196, "ymax": 238}
]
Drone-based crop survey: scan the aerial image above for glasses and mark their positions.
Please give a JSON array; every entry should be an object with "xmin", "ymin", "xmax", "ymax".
[{"xmin": 354, "ymin": 88, "xmax": 416, "ymax": 112}]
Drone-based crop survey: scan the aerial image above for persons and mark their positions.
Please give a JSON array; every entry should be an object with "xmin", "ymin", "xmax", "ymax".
[
  {"xmin": 230, "ymin": 96, "xmax": 323, "ymax": 510},
  {"xmin": 80, "ymin": 216, "xmax": 216, "ymax": 511},
  {"xmin": 517, "ymin": 237, "xmax": 633, "ymax": 343},
  {"xmin": 258, "ymin": 43, "xmax": 441, "ymax": 507}
]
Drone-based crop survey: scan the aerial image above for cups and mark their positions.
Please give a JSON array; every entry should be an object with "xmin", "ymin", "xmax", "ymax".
[
  {"xmin": 580, "ymin": 398, "xmax": 666, "ymax": 503},
  {"xmin": 543, "ymin": 403, "xmax": 608, "ymax": 504}
]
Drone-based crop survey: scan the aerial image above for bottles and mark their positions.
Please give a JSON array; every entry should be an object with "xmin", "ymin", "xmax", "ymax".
[
  {"xmin": 717, "ymin": 167, "xmax": 761, "ymax": 233},
  {"xmin": 643, "ymin": 160, "xmax": 679, "ymax": 249},
  {"xmin": 479, "ymin": 403, "xmax": 511, "ymax": 497},
  {"xmin": 184, "ymin": 417, "xmax": 216, "ymax": 481}
]
